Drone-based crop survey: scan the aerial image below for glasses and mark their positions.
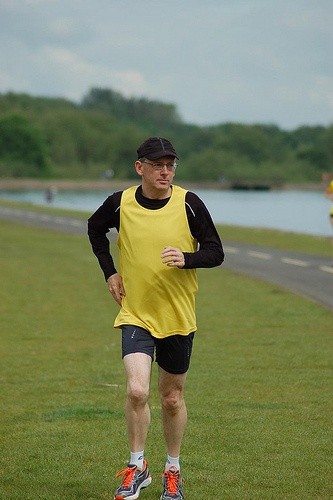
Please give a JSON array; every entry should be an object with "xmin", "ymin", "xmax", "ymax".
[{"xmin": 139, "ymin": 161, "xmax": 179, "ymax": 171}]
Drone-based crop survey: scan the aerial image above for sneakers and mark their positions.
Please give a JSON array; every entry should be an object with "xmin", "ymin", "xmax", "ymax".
[
  {"xmin": 113, "ymin": 460, "xmax": 152, "ymax": 500},
  {"xmin": 160, "ymin": 464, "xmax": 184, "ymax": 500}
]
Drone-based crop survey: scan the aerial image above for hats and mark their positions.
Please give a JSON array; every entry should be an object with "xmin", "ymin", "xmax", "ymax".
[{"xmin": 136, "ymin": 136, "xmax": 179, "ymax": 161}]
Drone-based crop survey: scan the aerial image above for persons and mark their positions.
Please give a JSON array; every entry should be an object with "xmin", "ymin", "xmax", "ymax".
[{"xmin": 87, "ymin": 138, "xmax": 226, "ymax": 494}]
